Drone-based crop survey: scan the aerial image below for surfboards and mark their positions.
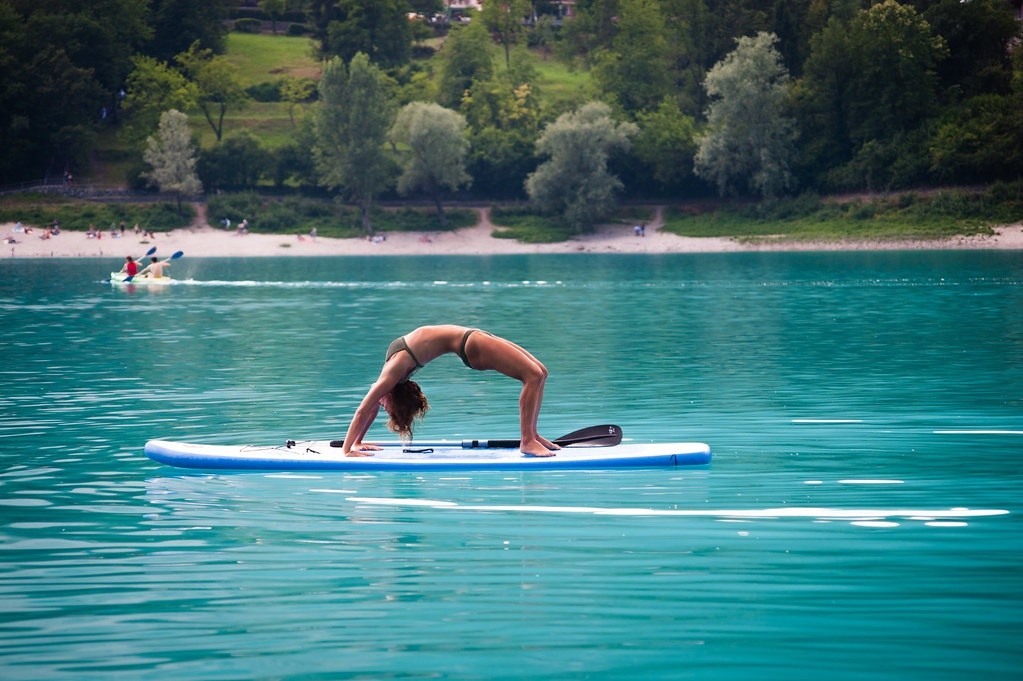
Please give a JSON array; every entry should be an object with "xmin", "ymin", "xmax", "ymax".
[{"xmin": 141, "ymin": 439, "xmax": 712, "ymax": 471}]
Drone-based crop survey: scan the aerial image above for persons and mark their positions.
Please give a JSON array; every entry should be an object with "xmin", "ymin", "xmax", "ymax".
[
  {"xmin": 119, "ymin": 256, "xmax": 143, "ymax": 275},
  {"xmin": 2, "ymin": 218, "xmax": 60, "ymax": 243},
  {"xmin": 342, "ymin": 323, "xmax": 560, "ymax": 458},
  {"xmin": 236, "ymin": 218, "xmax": 248, "ymax": 235},
  {"xmin": 634, "ymin": 223, "xmax": 645, "ymax": 236},
  {"xmin": 86, "ymin": 220, "xmax": 155, "ymax": 241},
  {"xmin": 142, "ymin": 256, "xmax": 170, "ymax": 278},
  {"xmin": 297, "ymin": 225, "xmax": 318, "ymax": 243}
]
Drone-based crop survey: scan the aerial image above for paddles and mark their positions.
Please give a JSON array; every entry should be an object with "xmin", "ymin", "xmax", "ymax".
[
  {"xmin": 104, "ymin": 246, "xmax": 157, "ymax": 282},
  {"xmin": 330, "ymin": 423, "xmax": 624, "ymax": 448},
  {"xmin": 122, "ymin": 250, "xmax": 184, "ymax": 282}
]
